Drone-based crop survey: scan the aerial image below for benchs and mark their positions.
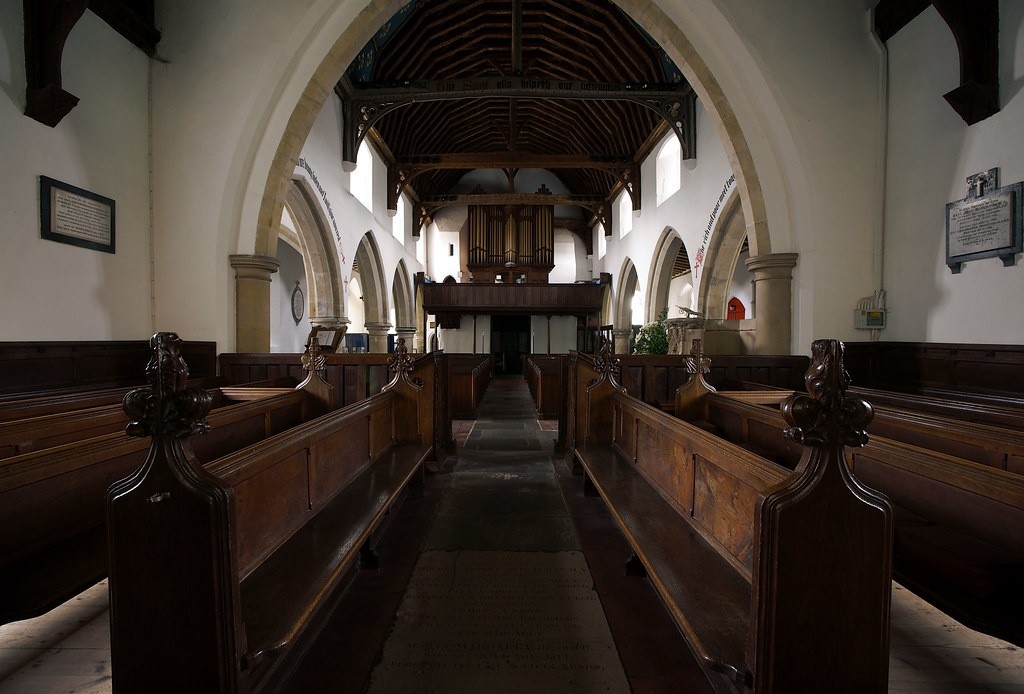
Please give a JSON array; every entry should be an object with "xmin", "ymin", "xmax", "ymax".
[
  {"xmin": 525, "ymin": 358, "xmax": 561, "ymax": 420},
  {"xmin": 570, "ymin": 337, "xmax": 895, "ymax": 694},
  {"xmin": 452, "ymin": 356, "xmax": 492, "ymax": 421},
  {"xmin": 0, "ymin": 334, "xmax": 335, "ymax": 629},
  {"xmin": 99, "ymin": 331, "xmax": 436, "ymax": 694},
  {"xmin": 0, "ymin": 389, "xmax": 223, "ymax": 459},
  {"xmin": 0, "ymin": 385, "xmax": 152, "ymax": 423},
  {"xmin": 849, "ymin": 384, "xmax": 1024, "ymax": 433},
  {"xmin": 793, "ymin": 390, "xmax": 1024, "ymax": 476},
  {"xmin": 673, "ymin": 339, "xmax": 1024, "ymax": 651}
]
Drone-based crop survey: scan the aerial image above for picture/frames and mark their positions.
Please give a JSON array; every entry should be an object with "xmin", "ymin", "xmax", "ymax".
[{"xmin": 39, "ymin": 174, "xmax": 116, "ymax": 255}]
